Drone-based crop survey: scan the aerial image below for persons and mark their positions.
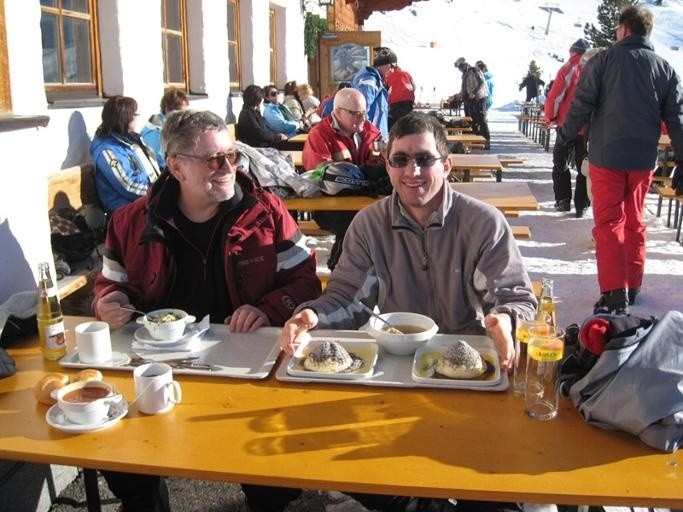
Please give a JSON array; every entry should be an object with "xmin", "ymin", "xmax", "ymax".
[
  {"xmin": 384, "ymin": 65, "xmax": 415, "ymax": 133},
  {"xmin": 555, "ymin": 5, "xmax": 683, "ymax": 316},
  {"xmin": 302, "ymin": 88, "xmax": 389, "ymax": 270},
  {"xmin": 89, "ymin": 107, "xmax": 323, "ymax": 511},
  {"xmin": 262, "ymin": 81, "xmax": 352, "ymax": 137},
  {"xmin": 351, "ymin": 48, "xmax": 397, "ymax": 144},
  {"xmin": 237, "ymin": 85, "xmax": 288, "ymax": 151},
  {"xmin": 543, "ymin": 37, "xmax": 592, "ymax": 218},
  {"xmin": 89, "ymin": 95, "xmax": 168, "ymax": 218},
  {"xmin": 447, "ymin": 57, "xmax": 494, "ymax": 151},
  {"xmin": 519, "ymin": 70, "xmax": 544, "ymax": 116},
  {"xmin": 139, "ymin": 87, "xmax": 192, "ymax": 173},
  {"xmin": 277, "ymin": 109, "xmax": 540, "ymax": 511}
]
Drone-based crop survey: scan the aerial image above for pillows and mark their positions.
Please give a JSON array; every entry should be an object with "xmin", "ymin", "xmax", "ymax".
[
  {"xmin": 319, "ymin": 161, "xmax": 369, "ymax": 196},
  {"xmin": 454, "ymin": 57, "xmax": 467, "ymax": 67}
]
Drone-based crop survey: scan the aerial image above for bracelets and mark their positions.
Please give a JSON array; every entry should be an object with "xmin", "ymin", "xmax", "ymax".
[
  {"xmin": 136, "ymin": 308, "xmax": 196, "ymax": 339},
  {"xmin": 365, "ymin": 311, "xmax": 439, "ymax": 358}
]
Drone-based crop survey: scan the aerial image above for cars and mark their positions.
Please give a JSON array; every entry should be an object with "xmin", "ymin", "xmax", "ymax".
[
  {"xmin": 510, "ymin": 311, "xmax": 566, "ymax": 420},
  {"xmin": 75, "ymin": 320, "xmax": 111, "ymax": 365},
  {"xmin": 132, "ymin": 362, "xmax": 181, "ymax": 417},
  {"xmin": 50, "ymin": 380, "xmax": 122, "ymax": 424}
]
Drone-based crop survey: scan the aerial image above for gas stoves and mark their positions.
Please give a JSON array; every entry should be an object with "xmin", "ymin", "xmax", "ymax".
[
  {"xmin": 432, "ymin": 340, "xmax": 486, "ymax": 380},
  {"xmin": 303, "ymin": 340, "xmax": 353, "ymax": 374}
]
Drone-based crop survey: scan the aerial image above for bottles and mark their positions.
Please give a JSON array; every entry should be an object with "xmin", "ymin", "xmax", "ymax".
[
  {"xmin": 569, "ymin": 38, "xmax": 590, "ymax": 53},
  {"xmin": 374, "ymin": 47, "xmax": 397, "ymax": 66}
]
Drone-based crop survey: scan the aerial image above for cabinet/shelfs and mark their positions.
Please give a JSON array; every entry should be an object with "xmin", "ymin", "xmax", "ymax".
[
  {"xmin": 534, "ymin": 275, "xmax": 556, "ymax": 326},
  {"xmin": 34, "ymin": 261, "xmax": 67, "ymax": 363}
]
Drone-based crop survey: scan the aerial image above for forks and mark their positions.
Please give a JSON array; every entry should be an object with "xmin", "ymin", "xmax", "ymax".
[{"xmin": 125, "ymin": 350, "xmax": 192, "ymax": 367}]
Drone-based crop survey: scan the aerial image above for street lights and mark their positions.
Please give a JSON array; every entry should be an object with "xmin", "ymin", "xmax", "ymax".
[{"xmin": 48, "ymin": 208, "xmax": 98, "ymax": 270}]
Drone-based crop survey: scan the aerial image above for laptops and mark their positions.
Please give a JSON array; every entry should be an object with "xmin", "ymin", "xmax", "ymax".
[
  {"xmin": 68, "ymin": 368, "xmax": 101, "ymax": 384},
  {"xmin": 34, "ymin": 373, "xmax": 69, "ymax": 405}
]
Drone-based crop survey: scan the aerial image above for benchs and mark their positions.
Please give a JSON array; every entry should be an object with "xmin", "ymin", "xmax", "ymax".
[{"xmin": 648, "ymin": 135, "xmax": 679, "ymax": 242}]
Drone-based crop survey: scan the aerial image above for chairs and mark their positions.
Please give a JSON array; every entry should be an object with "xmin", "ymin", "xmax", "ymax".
[
  {"xmin": 554, "ymin": 200, "xmax": 570, "ymax": 211},
  {"xmin": 592, "ymin": 288, "xmax": 629, "ymax": 315},
  {"xmin": 575, "ymin": 209, "xmax": 586, "ymax": 218},
  {"xmin": 628, "ymin": 288, "xmax": 641, "ymax": 306}
]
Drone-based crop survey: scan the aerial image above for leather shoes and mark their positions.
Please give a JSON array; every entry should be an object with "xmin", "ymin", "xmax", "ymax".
[
  {"xmin": 553, "ymin": 131, "xmax": 574, "ymax": 174},
  {"xmin": 670, "ymin": 164, "xmax": 682, "ymax": 196}
]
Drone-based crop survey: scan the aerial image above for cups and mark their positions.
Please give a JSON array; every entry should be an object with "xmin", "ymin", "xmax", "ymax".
[
  {"xmin": 390, "ymin": 64, "xmax": 398, "ymax": 70},
  {"xmin": 339, "ymin": 107, "xmax": 367, "ymax": 119},
  {"xmin": 270, "ymin": 91, "xmax": 279, "ymax": 96},
  {"xmin": 175, "ymin": 150, "xmax": 242, "ymax": 170},
  {"xmin": 385, "ymin": 153, "xmax": 441, "ymax": 168}
]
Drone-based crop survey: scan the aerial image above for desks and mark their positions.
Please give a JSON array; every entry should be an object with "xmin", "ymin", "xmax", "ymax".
[
  {"xmin": 263, "ymin": 108, "xmax": 543, "ymax": 314},
  {"xmin": 0, "ymin": 316, "xmax": 681, "ymax": 511},
  {"xmin": 517, "ymin": 113, "xmax": 561, "ymax": 153}
]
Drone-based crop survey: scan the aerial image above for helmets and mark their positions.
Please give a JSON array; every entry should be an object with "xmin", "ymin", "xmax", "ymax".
[
  {"xmin": 412, "ymin": 342, "xmax": 501, "ymax": 387},
  {"xmin": 284, "ymin": 336, "xmax": 380, "ymax": 382},
  {"xmin": 43, "ymin": 396, "xmax": 128, "ymax": 435},
  {"xmin": 133, "ymin": 326, "xmax": 192, "ymax": 345},
  {"xmin": 59, "ymin": 350, "xmax": 132, "ymax": 369}
]
